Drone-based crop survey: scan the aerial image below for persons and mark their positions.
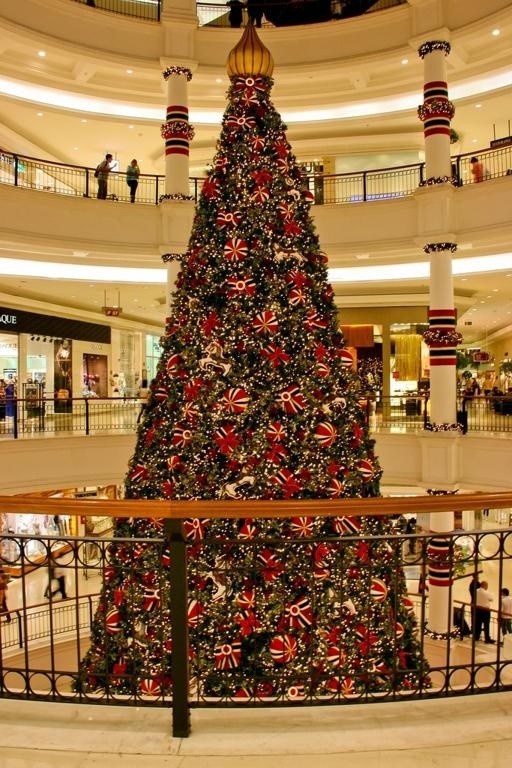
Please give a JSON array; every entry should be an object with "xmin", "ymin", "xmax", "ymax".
[
  {"xmin": 470, "ymin": 575, "xmax": 482, "ymax": 634},
  {"xmin": 501, "ymin": 588, "xmax": 512, "ymax": 644},
  {"xmin": 247, "ymin": 0, "xmax": 264, "ymax": 28},
  {"xmin": 136, "ymin": 379, "xmax": 150, "ymax": 423},
  {"xmin": 0, "ymin": 570, "xmax": 11, "ymax": 623},
  {"xmin": 475, "ymin": 581, "xmax": 496, "ymax": 644},
  {"xmin": 226, "ymin": 0, "xmax": 244, "ymax": 28},
  {"xmin": 126, "ymin": 158, "xmax": 140, "ymax": 203},
  {"xmin": 51, "ymin": 552, "xmax": 70, "ymax": 599},
  {"xmin": 470, "ymin": 157, "xmax": 488, "ymax": 183},
  {"xmin": 44, "ymin": 554, "xmax": 55, "ymax": 597},
  {"xmin": 97, "ymin": 154, "xmax": 117, "ymax": 199}
]
